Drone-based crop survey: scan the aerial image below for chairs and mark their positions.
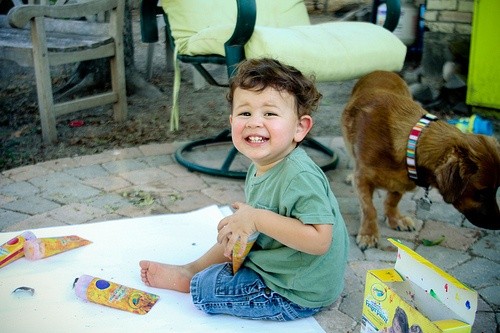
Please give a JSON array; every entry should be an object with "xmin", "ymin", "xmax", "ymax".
[
  {"xmin": 0, "ymin": 0, "xmax": 128, "ymax": 147},
  {"xmin": 140, "ymin": 1, "xmax": 407, "ymax": 179}
]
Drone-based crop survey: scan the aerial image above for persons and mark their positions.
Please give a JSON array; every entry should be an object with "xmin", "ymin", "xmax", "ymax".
[
  {"xmin": 379, "ymin": 308, "xmax": 422, "ymax": 333},
  {"xmin": 139, "ymin": 57, "xmax": 350, "ymax": 320}
]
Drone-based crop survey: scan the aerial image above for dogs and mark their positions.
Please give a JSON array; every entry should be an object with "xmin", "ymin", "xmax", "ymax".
[{"xmin": 338, "ymin": 66, "xmax": 498, "ymax": 257}]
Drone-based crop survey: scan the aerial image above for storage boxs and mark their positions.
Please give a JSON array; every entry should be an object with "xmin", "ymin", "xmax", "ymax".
[{"xmin": 359, "ymin": 236, "xmax": 479, "ymax": 333}]
